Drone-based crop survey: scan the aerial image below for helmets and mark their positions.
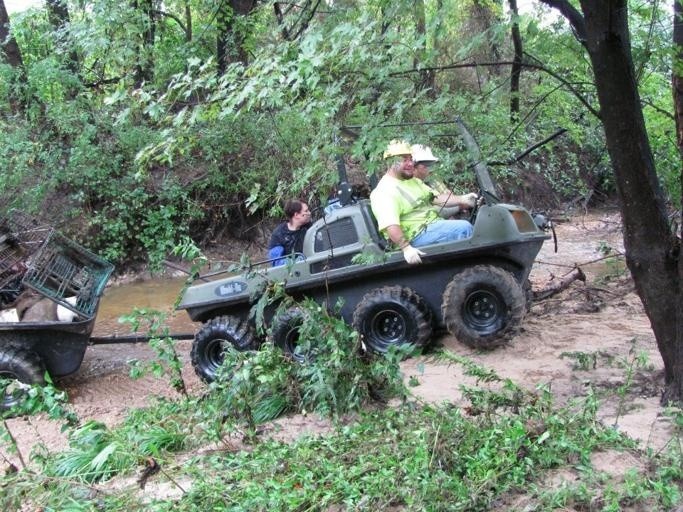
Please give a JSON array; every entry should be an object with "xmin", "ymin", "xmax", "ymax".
[
  {"xmin": 383, "ymin": 138, "xmax": 412, "ymax": 159},
  {"xmin": 411, "ymin": 143, "xmax": 440, "ymax": 164}
]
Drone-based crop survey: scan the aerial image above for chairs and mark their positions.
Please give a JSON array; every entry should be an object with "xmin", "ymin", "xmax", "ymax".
[{"xmin": 362, "ymin": 199, "xmax": 395, "ymax": 255}]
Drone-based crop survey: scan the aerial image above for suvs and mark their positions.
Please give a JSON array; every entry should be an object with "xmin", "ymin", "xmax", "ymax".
[{"xmin": 176, "ymin": 119, "xmax": 551, "ymax": 384}]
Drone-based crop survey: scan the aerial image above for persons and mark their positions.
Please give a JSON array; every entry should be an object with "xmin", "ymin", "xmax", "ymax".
[
  {"xmin": 367, "ymin": 141, "xmax": 483, "ymax": 265},
  {"xmin": 409, "ymin": 142, "xmax": 471, "ymax": 221},
  {"xmin": 268, "ymin": 197, "xmax": 313, "ymax": 267}
]
